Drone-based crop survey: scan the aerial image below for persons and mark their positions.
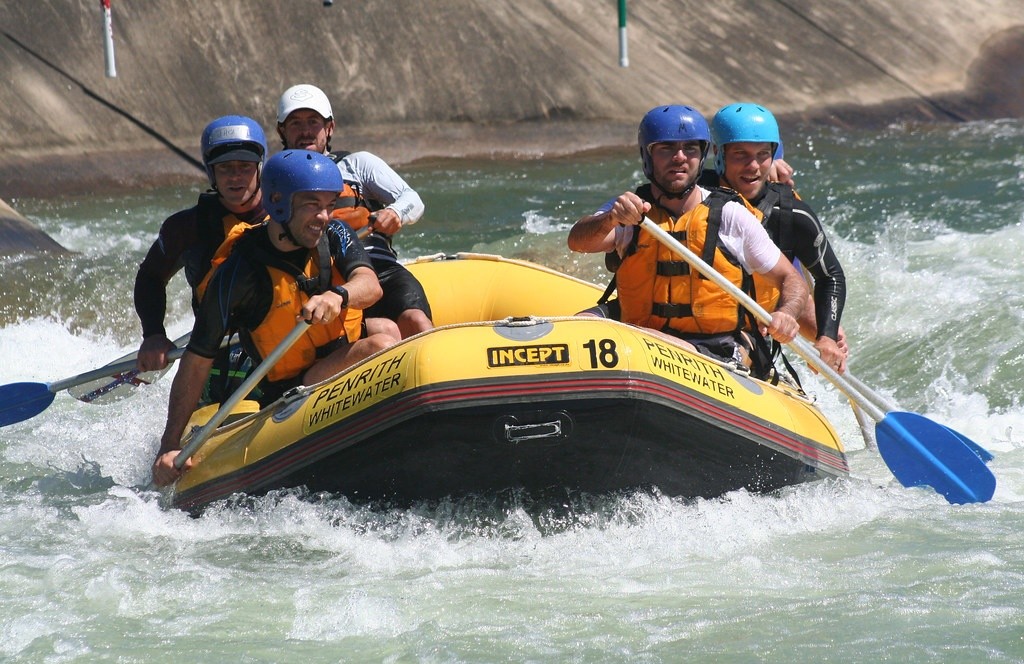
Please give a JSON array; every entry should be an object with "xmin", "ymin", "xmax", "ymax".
[
  {"xmin": 134, "ymin": 114, "xmax": 402, "ymax": 372},
  {"xmin": 276, "ymin": 84, "xmax": 435, "ymax": 342},
  {"xmin": 605, "ymin": 102, "xmax": 849, "ymax": 375},
  {"xmin": 567, "ymin": 104, "xmax": 809, "ymax": 362},
  {"xmin": 151, "ymin": 149, "xmax": 398, "ymax": 489}
]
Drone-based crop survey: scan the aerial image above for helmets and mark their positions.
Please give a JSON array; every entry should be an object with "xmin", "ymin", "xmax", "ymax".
[
  {"xmin": 260, "ymin": 148, "xmax": 344, "ymax": 224},
  {"xmin": 774, "ymin": 140, "xmax": 784, "ymax": 160},
  {"xmin": 709, "ymin": 102, "xmax": 781, "ymax": 178},
  {"xmin": 637, "ymin": 104, "xmax": 712, "ymax": 174},
  {"xmin": 275, "ymin": 84, "xmax": 334, "ymax": 123},
  {"xmin": 200, "ymin": 114, "xmax": 269, "ymax": 185}
]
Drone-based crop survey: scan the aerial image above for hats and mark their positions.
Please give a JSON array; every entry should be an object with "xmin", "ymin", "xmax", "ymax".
[{"xmin": 207, "ymin": 140, "xmax": 265, "ymax": 162}]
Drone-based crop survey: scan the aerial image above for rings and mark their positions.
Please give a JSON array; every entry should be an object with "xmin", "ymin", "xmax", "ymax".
[
  {"xmin": 834, "ymin": 361, "xmax": 840, "ymax": 366},
  {"xmin": 323, "ymin": 318, "xmax": 329, "ymax": 323}
]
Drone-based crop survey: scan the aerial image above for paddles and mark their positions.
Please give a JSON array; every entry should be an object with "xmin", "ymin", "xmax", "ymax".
[
  {"xmin": 618, "ymin": 198, "xmax": 997, "ymax": 506},
  {"xmin": 143, "ymin": 309, "xmax": 318, "ymax": 492},
  {"xmin": 842, "ymin": 362, "xmax": 874, "ymax": 447},
  {"xmin": 67, "ymin": 212, "xmax": 380, "ymax": 405},
  {"xmin": 0, "ymin": 332, "xmax": 241, "ymax": 428},
  {"xmin": 794, "ymin": 333, "xmax": 995, "ymax": 463}
]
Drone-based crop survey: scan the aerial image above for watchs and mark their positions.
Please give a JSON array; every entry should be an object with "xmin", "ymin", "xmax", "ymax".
[{"xmin": 331, "ymin": 286, "xmax": 348, "ymax": 309}]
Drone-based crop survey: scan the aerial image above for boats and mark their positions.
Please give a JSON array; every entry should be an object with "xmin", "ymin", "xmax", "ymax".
[{"xmin": 159, "ymin": 251, "xmax": 850, "ymax": 537}]
